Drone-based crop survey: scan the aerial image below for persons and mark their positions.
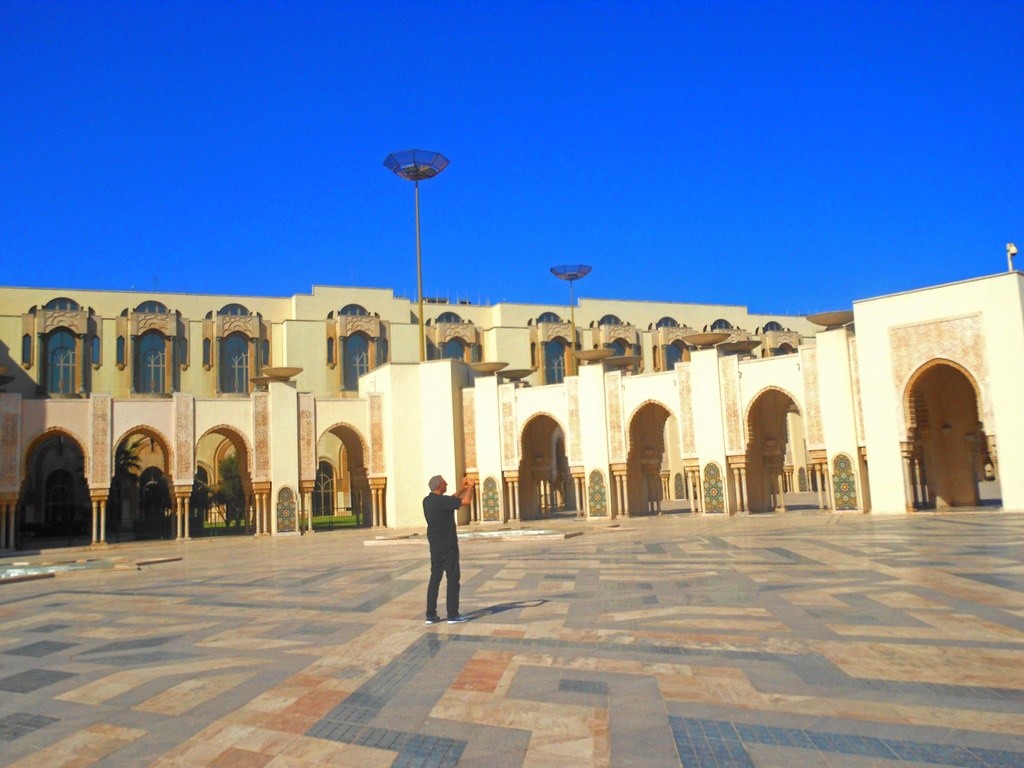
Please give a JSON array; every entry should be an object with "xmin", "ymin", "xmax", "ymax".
[{"xmin": 423, "ymin": 475, "xmax": 476, "ymax": 625}]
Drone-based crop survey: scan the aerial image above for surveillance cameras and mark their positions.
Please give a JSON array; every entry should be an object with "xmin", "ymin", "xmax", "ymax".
[{"xmin": 1009, "ymin": 246, "xmax": 1018, "ymax": 256}]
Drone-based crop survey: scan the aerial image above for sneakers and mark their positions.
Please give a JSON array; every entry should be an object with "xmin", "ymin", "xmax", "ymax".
[
  {"xmin": 447, "ymin": 615, "xmax": 469, "ymax": 624},
  {"xmin": 426, "ymin": 617, "xmax": 440, "ymax": 624}
]
